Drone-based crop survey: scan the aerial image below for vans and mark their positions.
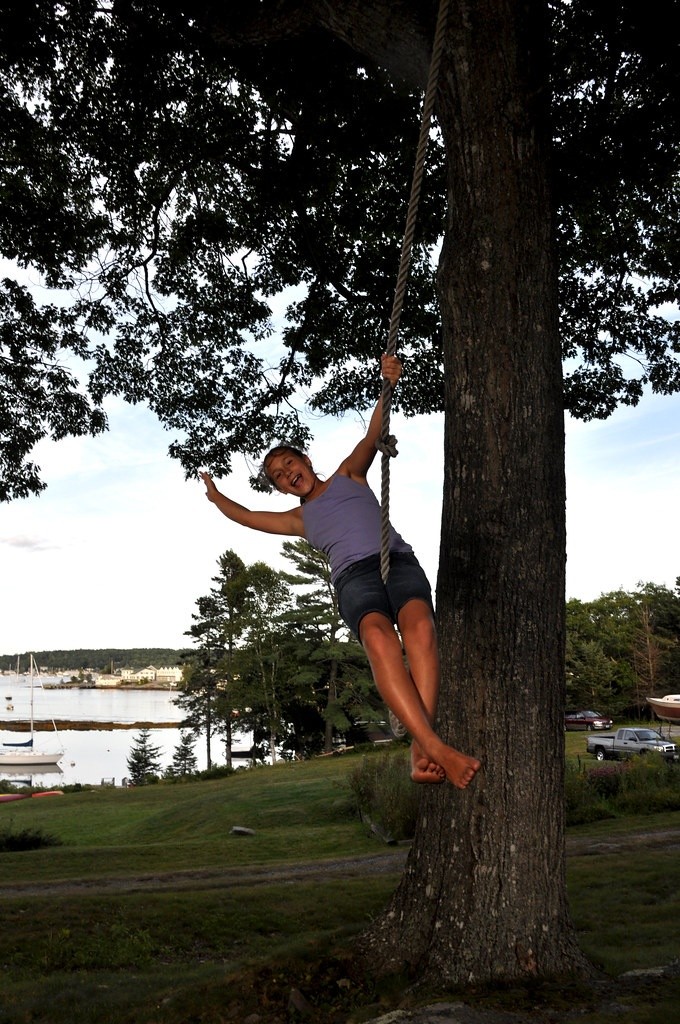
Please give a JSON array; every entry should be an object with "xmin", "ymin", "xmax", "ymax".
[{"xmin": 564, "ymin": 707, "xmax": 613, "ymax": 731}]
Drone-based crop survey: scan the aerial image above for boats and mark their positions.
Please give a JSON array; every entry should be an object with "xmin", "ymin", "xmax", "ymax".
[
  {"xmin": 646, "ymin": 694, "xmax": 680, "ymax": 725},
  {"xmin": 229, "ymin": 748, "xmax": 258, "ymax": 761}
]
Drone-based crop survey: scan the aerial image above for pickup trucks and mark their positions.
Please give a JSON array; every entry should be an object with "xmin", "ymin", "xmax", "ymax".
[{"xmin": 586, "ymin": 728, "xmax": 677, "ymax": 762}]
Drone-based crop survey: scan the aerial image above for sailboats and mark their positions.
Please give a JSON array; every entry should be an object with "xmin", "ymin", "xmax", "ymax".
[
  {"xmin": 0, "ymin": 764, "xmax": 65, "ymax": 787},
  {"xmin": 0, "ymin": 654, "xmax": 64, "ymax": 765},
  {"xmin": 169, "ymin": 684, "xmax": 177, "ymax": 703},
  {"xmin": 12, "ymin": 655, "xmax": 26, "ymax": 683}
]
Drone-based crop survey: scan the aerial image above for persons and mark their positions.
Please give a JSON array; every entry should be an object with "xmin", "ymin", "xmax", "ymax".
[{"xmin": 200, "ymin": 355, "xmax": 480, "ymax": 789}]
